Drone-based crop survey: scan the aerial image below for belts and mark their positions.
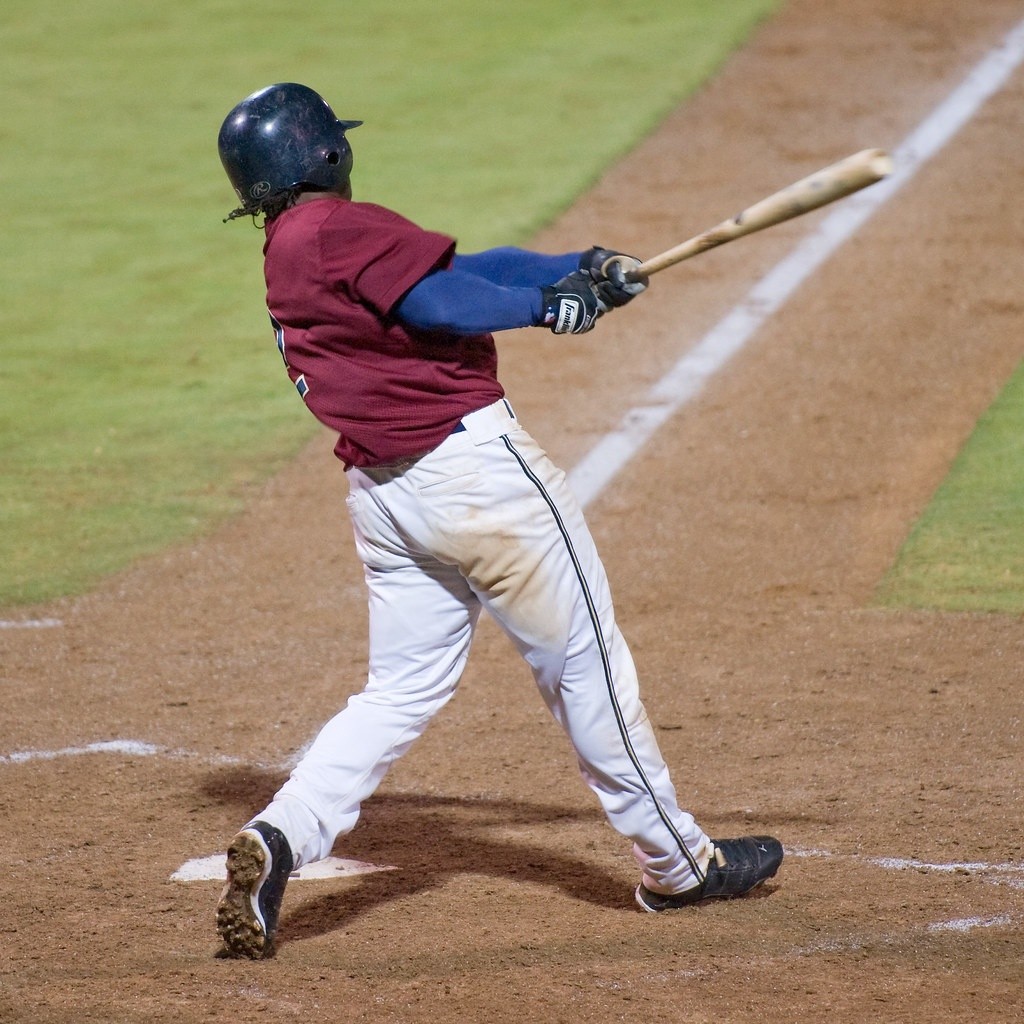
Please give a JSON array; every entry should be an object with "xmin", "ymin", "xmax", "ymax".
[{"xmin": 450, "ymin": 400, "xmax": 515, "ymax": 434}]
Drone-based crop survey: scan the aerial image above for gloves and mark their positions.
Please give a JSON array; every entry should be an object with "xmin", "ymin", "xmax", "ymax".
[
  {"xmin": 580, "ymin": 246, "xmax": 650, "ymax": 307},
  {"xmin": 538, "ymin": 281, "xmax": 613, "ymax": 336}
]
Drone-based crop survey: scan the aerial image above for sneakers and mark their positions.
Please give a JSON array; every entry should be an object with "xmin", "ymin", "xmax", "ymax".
[
  {"xmin": 635, "ymin": 835, "xmax": 784, "ymax": 913},
  {"xmin": 215, "ymin": 821, "xmax": 294, "ymax": 959}
]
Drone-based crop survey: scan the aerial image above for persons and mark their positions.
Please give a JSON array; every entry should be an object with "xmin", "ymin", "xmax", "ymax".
[{"xmin": 218, "ymin": 81, "xmax": 786, "ymax": 957}]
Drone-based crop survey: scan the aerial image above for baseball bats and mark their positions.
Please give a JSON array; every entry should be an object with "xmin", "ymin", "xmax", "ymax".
[{"xmin": 624, "ymin": 150, "xmax": 896, "ymax": 276}]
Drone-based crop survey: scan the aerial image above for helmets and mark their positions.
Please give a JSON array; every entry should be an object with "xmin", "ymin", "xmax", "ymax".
[{"xmin": 217, "ymin": 82, "xmax": 364, "ymax": 203}]
